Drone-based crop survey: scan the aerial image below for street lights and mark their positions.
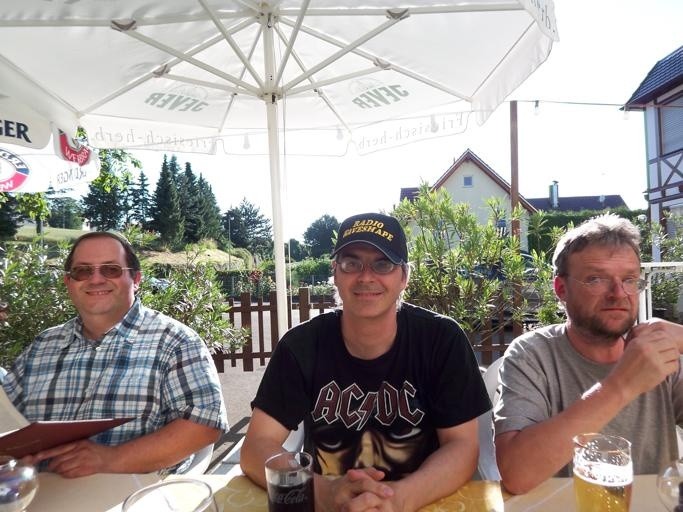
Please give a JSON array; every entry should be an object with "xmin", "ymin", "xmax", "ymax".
[{"xmin": 228, "ymin": 215, "xmax": 234, "ymax": 270}]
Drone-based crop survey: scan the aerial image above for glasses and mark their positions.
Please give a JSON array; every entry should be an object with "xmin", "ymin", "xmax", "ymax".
[
  {"xmin": 335, "ymin": 259, "xmax": 395, "ymax": 273},
  {"xmin": 66, "ymin": 263, "xmax": 134, "ymax": 279},
  {"xmin": 571, "ymin": 276, "xmax": 647, "ymax": 295}
]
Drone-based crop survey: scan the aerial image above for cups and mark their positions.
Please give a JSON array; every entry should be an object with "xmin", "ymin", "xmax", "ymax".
[
  {"xmin": 264, "ymin": 451, "xmax": 313, "ymax": 510},
  {"xmin": 120, "ymin": 478, "xmax": 219, "ymax": 512},
  {"xmin": 571, "ymin": 433, "xmax": 634, "ymax": 512}
]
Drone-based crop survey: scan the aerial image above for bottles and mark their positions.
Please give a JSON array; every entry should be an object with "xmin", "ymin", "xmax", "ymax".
[
  {"xmin": 0, "ymin": 458, "xmax": 39, "ymax": 510},
  {"xmin": 656, "ymin": 455, "xmax": 683, "ymax": 512}
]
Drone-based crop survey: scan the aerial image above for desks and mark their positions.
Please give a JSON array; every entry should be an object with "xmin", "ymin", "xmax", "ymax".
[{"xmin": 2, "ymin": 474, "xmax": 683, "ymax": 512}]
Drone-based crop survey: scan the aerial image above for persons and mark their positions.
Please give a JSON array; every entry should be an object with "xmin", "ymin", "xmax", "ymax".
[
  {"xmin": 0, "ymin": 231, "xmax": 230, "ymax": 479},
  {"xmin": 492, "ymin": 213, "xmax": 683, "ymax": 495},
  {"xmin": 240, "ymin": 213, "xmax": 493, "ymax": 512}
]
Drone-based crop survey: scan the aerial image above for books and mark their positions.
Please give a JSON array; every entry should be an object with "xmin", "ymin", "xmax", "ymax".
[{"xmin": 0, "ymin": 384, "xmax": 137, "ymax": 458}]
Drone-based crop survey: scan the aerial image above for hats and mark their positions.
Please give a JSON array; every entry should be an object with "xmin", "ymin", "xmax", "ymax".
[{"xmin": 329, "ymin": 214, "xmax": 408, "ymax": 264}]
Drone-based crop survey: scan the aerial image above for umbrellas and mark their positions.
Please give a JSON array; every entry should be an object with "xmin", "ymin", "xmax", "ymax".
[{"xmin": 0, "ymin": 1, "xmax": 558, "ymax": 341}]
